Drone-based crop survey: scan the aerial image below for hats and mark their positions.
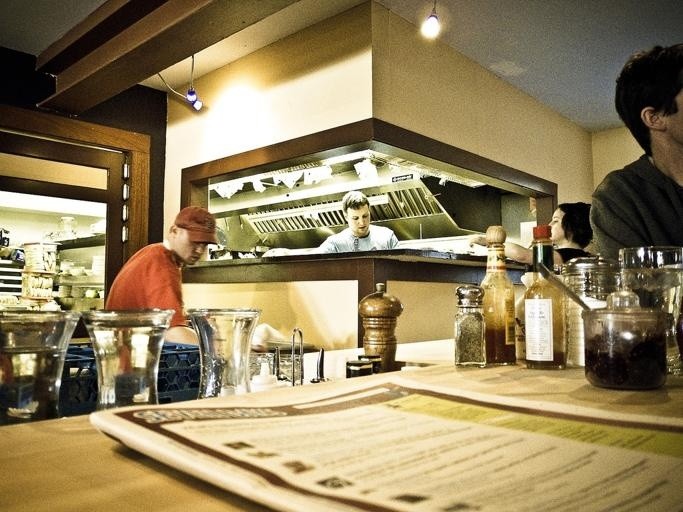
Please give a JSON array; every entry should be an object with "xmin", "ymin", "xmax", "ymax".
[{"xmin": 175, "ymin": 206, "xmax": 216, "ymax": 245}]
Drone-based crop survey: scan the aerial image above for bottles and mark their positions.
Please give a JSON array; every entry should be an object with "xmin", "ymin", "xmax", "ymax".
[
  {"xmin": 452, "ymin": 284, "xmax": 487, "ymax": 368},
  {"xmin": 578, "ymin": 290, "xmax": 674, "ymax": 391},
  {"xmin": 560, "ymin": 253, "xmax": 615, "ymax": 370},
  {"xmin": 477, "ymin": 222, "xmax": 517, "ymax": 367},
  {"xmin": 521, "ymin": 222, "xmax": 569, "ymax": 370}
]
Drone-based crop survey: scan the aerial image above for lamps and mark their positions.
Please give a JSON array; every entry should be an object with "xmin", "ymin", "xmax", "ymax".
[
  {"xmin": 159, "ymin": 55, "xmax": 202, "ymax": 110},
  {"xmin": 421, "ymin": 2, "xmax": 441, "ymax": 40}
]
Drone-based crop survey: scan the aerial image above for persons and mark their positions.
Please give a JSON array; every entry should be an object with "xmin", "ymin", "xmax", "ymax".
[
  {"xmin": 586, "ymin": 42, "xmax": 681, "ymax": 293},
  {"xmin": 467, "ymin": 201, "xmax": 599, "ymax": 280},
  {"xmin": 104, "ymin": 206, "xmax": 220, "ymax": 349},
  {"xmin": 317, "ymin": 191, "xmax": 401, "ymax": 254}
]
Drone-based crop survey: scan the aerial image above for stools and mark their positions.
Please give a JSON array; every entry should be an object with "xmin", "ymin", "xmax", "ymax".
[{"xmin": 250, "ymin": 340, "xmax": 320, "ymax": 383}]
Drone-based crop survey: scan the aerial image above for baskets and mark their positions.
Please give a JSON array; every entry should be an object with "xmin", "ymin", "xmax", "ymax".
[{"xmin": 45, "ymin": 341, "xmax": 225, "ymax": 419}]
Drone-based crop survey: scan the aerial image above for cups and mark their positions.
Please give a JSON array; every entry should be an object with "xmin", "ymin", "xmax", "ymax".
[
  {"xmin": 78, "ymin": 307, "xmax": 176, "ymax": 408},
  {"xmin": 186, "ymin": 306, "xmax": 262, "ymax": 400},
  {"xmin": 0, "ymin": 307, "xmax": 81, "ymax": 419},
  {"xmin": 248, "ymin": 351, "xmax": 303, "ymax": 389},
  {"xmin": 616, "ymin": 245, "xmax": 683, "ymax": 376}
]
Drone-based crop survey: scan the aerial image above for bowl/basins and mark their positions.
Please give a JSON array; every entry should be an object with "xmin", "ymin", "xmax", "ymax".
[{"xmin": 58, "ymin": 255, "xmax": 105, "ymax": 277}]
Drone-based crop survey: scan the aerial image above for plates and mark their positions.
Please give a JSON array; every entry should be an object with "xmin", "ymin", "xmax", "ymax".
[{"xmin": 56, "ymin": 285, "xmax": 104, "ymax": 299}]
